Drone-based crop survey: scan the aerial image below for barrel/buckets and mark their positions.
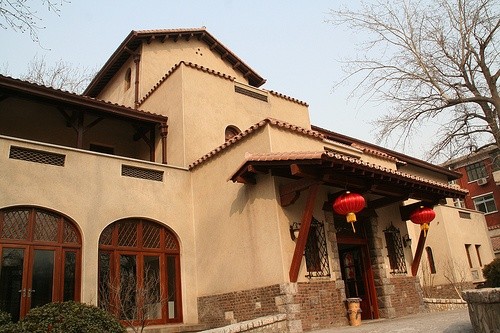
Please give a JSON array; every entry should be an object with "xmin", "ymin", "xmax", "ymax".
[{"xmin": 347, "ymin": 297, "xmax": 362, "ymax": 326}]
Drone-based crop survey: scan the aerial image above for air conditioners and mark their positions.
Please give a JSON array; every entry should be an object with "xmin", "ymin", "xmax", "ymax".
[{"xmin": 476, "ymin": 177, "xmax": 488, "ymax": 186}]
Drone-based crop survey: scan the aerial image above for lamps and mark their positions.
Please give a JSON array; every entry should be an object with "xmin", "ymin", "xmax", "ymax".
[
  {"xmin": 289, "ymin": 221, "xmax": 300, "ymax": 241},
  {"xmin": 402, "ymin": 233, "xmax": 412, "ymax": 250}
]
